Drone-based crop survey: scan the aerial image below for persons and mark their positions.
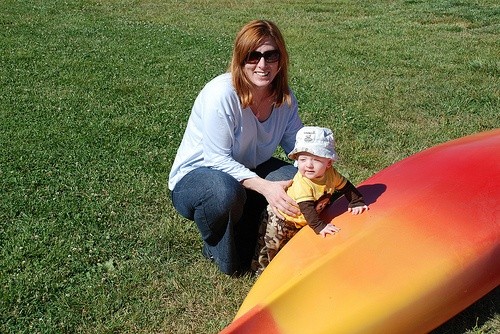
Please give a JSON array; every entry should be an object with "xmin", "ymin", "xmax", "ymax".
[
  {"xmin": 165, "ymin": 22, "xmax": 306, "ymax": 277},
  {"xmin": 256, "ymin": 126, "xmax": 368, "ymax": 275}
]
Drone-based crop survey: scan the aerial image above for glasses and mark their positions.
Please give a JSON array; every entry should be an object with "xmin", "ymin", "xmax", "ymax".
[{"xmin": 247, "ymin": 47, "xmax": 282, "ymax": 64}]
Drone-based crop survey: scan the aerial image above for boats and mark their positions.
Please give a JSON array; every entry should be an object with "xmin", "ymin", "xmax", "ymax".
[{"xmin": 217, "ymin": 129, "xmax": 500, "ymax": 334}]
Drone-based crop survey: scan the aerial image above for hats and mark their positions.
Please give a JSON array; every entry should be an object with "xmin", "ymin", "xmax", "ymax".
[{"xmin": 286, "ymin": 125, "xmax": 338, "ymax": 160}]
